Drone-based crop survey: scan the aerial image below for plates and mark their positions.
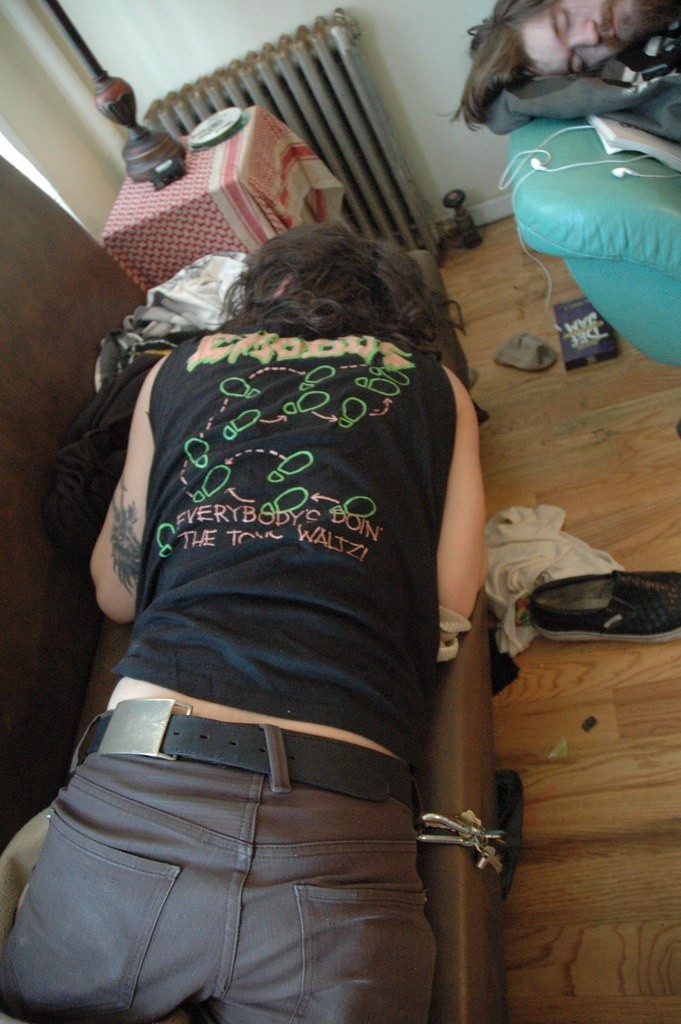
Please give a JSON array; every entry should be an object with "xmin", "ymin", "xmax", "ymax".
[{"xmin": 187, "ymin": 107, "xmax": 244, "ymax": 147}]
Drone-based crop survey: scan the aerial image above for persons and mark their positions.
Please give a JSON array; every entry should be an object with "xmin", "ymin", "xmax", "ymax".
[
  {"xmin": 444, "ymin": 0, "xmax": 681, "ymax": 133},
  {"xmin": 0, "ymin": 222, "xmax": 486, "ymax": 1024}
]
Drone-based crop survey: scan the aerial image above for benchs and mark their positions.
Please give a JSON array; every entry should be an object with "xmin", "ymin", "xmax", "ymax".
[{"xmin": 0, "ymin": 152, "xmax": 500, "ymax": 1024}]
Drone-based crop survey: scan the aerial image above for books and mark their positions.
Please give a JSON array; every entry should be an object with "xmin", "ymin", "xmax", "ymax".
[{"xmin": 584, "ymin": 114, "xmax": 681, "ymax": 173}]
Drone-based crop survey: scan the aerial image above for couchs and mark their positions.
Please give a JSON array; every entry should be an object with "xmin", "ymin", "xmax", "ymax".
[{"xmin": 511, "ymin": 113, "xmax": 681, "ymax": 366}]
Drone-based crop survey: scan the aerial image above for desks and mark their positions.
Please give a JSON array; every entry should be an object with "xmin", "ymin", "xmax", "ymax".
[{"xmin": 100, "ymin": 106, "xmax": 347, "ymax": 295}]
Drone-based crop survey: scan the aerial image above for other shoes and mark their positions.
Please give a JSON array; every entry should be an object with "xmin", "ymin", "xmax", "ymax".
[{"xmin": 526, "ymin": 569, "xmax": 681, "ymax": 642}]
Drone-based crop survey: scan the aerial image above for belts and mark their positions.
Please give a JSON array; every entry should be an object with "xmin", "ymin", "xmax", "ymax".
[{"xmin": 87, "ymin": 709, "xmax": 413, "ymax": 806}]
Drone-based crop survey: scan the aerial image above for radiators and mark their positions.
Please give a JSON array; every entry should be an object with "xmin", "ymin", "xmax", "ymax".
[{"xmin": 143, "ymin": 9, "xmax": 483, "ymax": 267}]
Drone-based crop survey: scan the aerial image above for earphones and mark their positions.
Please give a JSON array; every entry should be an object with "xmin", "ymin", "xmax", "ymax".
[
  {"xmin": 531, "ymin": 158, "xmax": 547, "ymax": 171},
  {"xmin": 612, "ymin": 167, "xmax": 639, "ymax": 178}
]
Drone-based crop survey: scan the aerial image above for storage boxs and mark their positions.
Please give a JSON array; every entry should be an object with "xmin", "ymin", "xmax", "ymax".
[{"xmin": 553, "ymin": 297, "xmax": 617, "ymax": 370}]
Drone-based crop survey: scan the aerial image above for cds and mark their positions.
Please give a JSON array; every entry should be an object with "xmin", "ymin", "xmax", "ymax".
[{"xmin": 188, "ymin": 107, "xmax": 249, "ymax": 152}]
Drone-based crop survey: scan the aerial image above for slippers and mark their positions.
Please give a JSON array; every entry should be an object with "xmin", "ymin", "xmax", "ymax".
[{"xmin": 493, "ymin": 333, "xmax": 557, "ymax": 370}]
